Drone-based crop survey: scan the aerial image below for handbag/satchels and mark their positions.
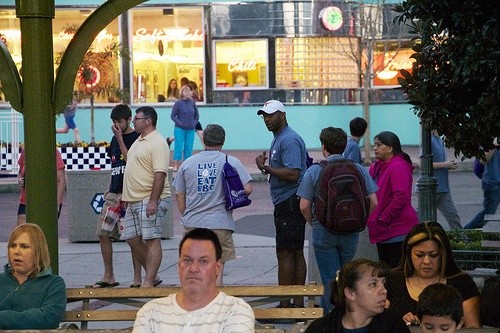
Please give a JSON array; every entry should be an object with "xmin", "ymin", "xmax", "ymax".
[
  {"xmin": 222, "ymin": 156, "xmax": 252, "ymax": 210},
  {"xmin": 306, "ymin": 148, "xmax": 314, "ymax": 169},
  {"xmin": 474, "ymin": 150, "xmax": 485, "ymax": 179}
]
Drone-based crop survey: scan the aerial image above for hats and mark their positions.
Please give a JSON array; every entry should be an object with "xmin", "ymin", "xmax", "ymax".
[{"xmin": 257, "ymin": 100, "xmax": 286, "ymax": 114}]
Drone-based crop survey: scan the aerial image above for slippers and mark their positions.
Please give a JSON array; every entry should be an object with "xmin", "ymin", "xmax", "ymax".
[
  {"xmin": 129, "ymin": 283, "xmax": 141, "ymax": 288},
  {"xmin": 153, "ymin": 279, "xmax": 163, "ymax": 288},
  {"xmin": 91, "ymin": 279, "xmax": 119, "ymax": 289}
]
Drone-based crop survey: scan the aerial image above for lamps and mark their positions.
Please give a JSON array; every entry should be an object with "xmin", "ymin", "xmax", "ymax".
[{"xmin": 163, "ymin": 8, "xmax": 190, "ymax": 36}]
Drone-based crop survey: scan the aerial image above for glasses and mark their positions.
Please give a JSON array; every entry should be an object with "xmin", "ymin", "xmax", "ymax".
[
  {"xmin": 135, "ymin": 115, "xmax": 148, "ymax": 121},
  {"xmin": 260, "ymin": 115, "xmax": 275, "ymax": 119}
]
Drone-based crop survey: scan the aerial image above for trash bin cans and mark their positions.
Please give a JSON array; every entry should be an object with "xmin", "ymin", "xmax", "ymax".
[
  {"xmin": 119, "ymin": 167, "xmax": 174, "ymax": 241},
  {"xmin": 64, "ymin": 170, "xmax": 117, "ymax": 242}
]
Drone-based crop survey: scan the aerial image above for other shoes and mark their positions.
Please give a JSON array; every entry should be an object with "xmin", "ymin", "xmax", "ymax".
[
  {"xmin": 166, "ymin": 137, "xmax": 172, "ymax": 149},
  {"xmin": 255, "ymin": 305, "xmax": 305, "ymax": 324}
]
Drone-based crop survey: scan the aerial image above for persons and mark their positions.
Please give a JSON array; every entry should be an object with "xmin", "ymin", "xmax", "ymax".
[
  {"xmin": 122, "ymin": 107, "xmax": 174, "ymax": 288},
  {"xmin": 132, "ymin": 228, "xmax": 255, "ymax": 333},
  {"xmin": 296, "ymin": 126, "xmax": 379, "ymax": 314},
  {"xmin": 56, "ymin": 96, "xmax": 81, "ymax": 143},
  {"xmin": 172, "ymin": 124, "xmax": 253, "ymax": 286},
  {"xmin": 342, "ymin": 117, "xmax": 368, "ymax": 165},
  {"xmin": 0, "ymin": 223, "xmax": 68, "ymax": 330},
  {"xmin": 90, "ymin": 104, "xmax": 163, "ymax": 289},
  {"xmin": 415, "ymin": 126, "xmax": 462, "ymax": 232},
  {"xmin": 256, "ymin": 100, "xmax": 309, "ymax": 308},
  {"xmin": 162, "ymin": 77, "xmax": 209, "ymax": 170},
  {"xmin": 463, "ymin": 141, "xmax": 500, "ymax": 230},
  {"xmin": 16, "ymin": 145, "xmax": 65, "ymax": 226},
  {"xmin": 367, "ymin": 132, "xmax": 420, "ymax": 267},
  {"xmin": 303, "ymin": 222, "xmax": 481, "ymax": 333}
]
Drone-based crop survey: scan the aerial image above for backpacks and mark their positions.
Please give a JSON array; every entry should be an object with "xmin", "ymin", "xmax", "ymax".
[{"xmin": 310, "ymin": 159, "xmax": 370, "ymax": 234}]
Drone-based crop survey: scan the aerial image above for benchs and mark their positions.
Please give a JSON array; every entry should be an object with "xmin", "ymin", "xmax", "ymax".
[{"xmin": 62, "ymin": 283, "xmax": 325, "ymax": 333}]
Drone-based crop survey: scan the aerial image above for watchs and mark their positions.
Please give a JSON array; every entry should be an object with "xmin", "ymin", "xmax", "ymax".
[{"xmin": 261, "ymin": 166, "xmax": 268, "ymax": 175}]
